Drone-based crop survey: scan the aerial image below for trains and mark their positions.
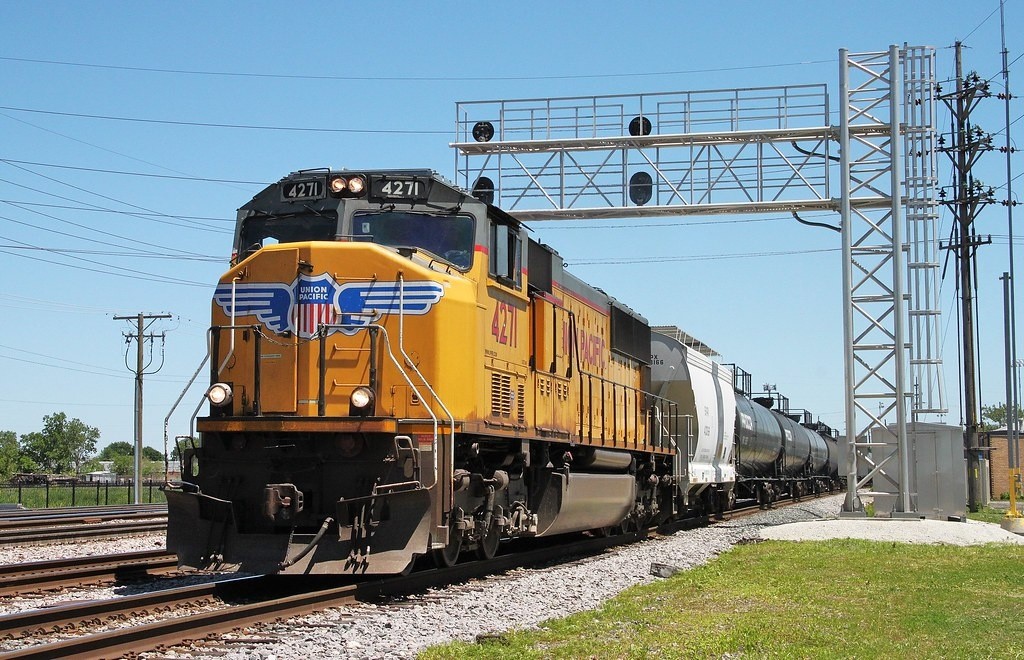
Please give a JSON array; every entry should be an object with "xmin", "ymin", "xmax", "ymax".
[{"xmin": 158, "ymin": 166, "xmax": 838, "ymax": 579}]
